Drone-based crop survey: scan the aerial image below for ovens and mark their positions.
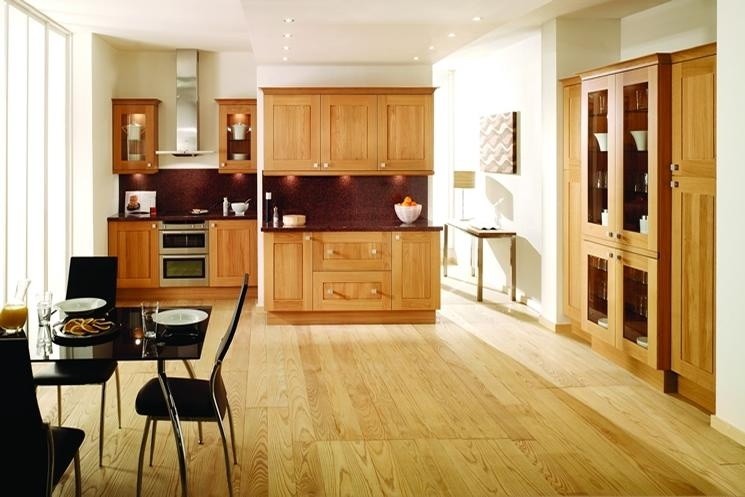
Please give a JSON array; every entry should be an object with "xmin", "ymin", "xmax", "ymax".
[{"xmin": 157, "ymin": 229, "xmax": 209, "ymax": 286}]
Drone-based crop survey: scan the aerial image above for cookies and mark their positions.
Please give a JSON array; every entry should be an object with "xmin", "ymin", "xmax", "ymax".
[{"xmin": 62, "ymin": 319, "xmax": 114, "ymax": 334}]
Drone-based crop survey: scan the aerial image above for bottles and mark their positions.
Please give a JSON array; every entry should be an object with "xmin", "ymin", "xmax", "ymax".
[
  {"xmin": 593, "ymin": 167, "xmax": 648, "ymax": 233},
  {"xmin": 273, "ymin": 205, "xmax": 279, "ymax": 228},
  {"xmin": 126, "ymin": 119, "xmax": 143, "ymax": 161},
  {"xmin": 222, "ymin": 197, "xmax": 228, "ymax": 216}
]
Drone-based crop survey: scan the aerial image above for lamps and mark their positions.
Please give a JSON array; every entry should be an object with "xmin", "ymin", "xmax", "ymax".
[{"xmin": 453, "ymin": 170, "xmax": 475, "ymax": 223}]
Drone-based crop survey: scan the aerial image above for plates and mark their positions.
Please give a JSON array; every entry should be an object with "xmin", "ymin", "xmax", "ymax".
[
  {"xmin": 597, "ymin": 319, "xmax": 609, "ymax": 326},
  {"xmin": 155, "ymin": 308, "xmax": 210, "ymax": 325},
  {"xmin": 125, "ymin": 202, "xmax": 140, "ymax": 210},
  {"xmin": 55, "ymin": 297, "xmax": 106, "ymax": 315},
  {"xmin": 637, "ymin": 335, "xmax": 647, "ymax": 347},
  {"xmin": 52, "ymin": 319, "xmax": 121, "ymax": 340}
]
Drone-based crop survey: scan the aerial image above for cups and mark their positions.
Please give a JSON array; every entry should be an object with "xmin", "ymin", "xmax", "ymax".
[
  {"xmin": 591, "ymin": 256, "xmax": 648, "ymax": 317},
  {"xmin": 231, "ymin": 153, "xmax": 249, "ymax": 160},
  {"xmin": 0, "ymin": 280, "xmax": 29, "ymax": 333},
  {"xmin": 36, "ymin": 302, "xmax": 50, "ymax": 326},
  {"xmin": 191, "ymin": 207, "xmax": 208, "ymax": 215},
  {"xmin": 141, "ymin": 301, "xmax": 159, "ymax": 339},
  {"xmin": 636, "ymin": 89, "xmax": 648, "ymax": 112},
  {"xmin": 593, "ymin": 93, "xmax": 606, "ymax": 114},
  {"xmin": 150, "ymin": 207, "xmax": 158, "ymax": 216}
]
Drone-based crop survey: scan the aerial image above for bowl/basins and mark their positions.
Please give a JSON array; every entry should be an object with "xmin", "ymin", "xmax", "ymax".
[
  {"xmin": 592, "ymin": 132, "xmax": 608, "ymax": 151},
  {"xmin": 231, "ymin": 203, "xmax": 249, "ymax": 216},
  {"xmin": 394, "ymin": 203, "xmax": 422, "ymax": 224},
  {"xmin": 630, "ymin": 130, "xmax": 648, "ymax": 151},
  {"xmin": 282, "ymin": 215, "xmax": 306, "ymax": 226}
]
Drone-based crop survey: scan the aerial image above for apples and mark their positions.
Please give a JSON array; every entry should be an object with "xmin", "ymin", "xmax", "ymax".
[{"xmin": 402, "ymin": 196, "xmax": 417, "ymax": 207}]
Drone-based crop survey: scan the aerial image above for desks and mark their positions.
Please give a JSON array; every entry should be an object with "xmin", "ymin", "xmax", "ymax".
[{"xmin": 444, "ymin": 221, "xmax": 517, "ymax": 303}]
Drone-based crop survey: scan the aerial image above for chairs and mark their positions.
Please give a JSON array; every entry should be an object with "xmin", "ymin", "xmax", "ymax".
[
  {"xmin": 55, "ymin": 256, "xmax": 124, "ymax": 428},
  {"xmin": 135, "ymin": 272, "xmax": 250, "ymax": 496},
  {"xmin": 1, "ymin": 337, "xmax": 85, "ymax": 497}
]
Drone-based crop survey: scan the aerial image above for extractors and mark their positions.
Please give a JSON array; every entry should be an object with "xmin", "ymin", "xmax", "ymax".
[{"xmin": 155, "ymin": 48, "xmax": 216, "ymax": 157}]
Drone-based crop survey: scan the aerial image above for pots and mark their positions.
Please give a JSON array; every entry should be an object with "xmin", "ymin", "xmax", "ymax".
[{"xmin": 227, "ymin": 120, "xmax": 250, "ymax": 140}]
